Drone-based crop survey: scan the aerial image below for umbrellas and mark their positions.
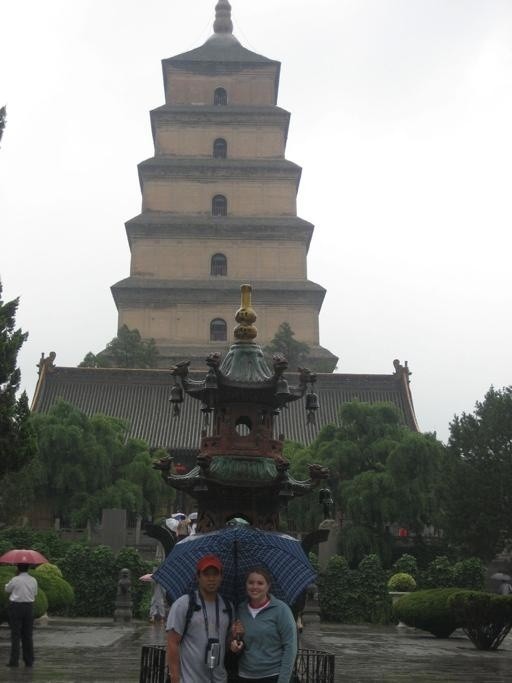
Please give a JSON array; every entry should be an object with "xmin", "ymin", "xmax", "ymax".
[
  {"xmin": 138, "ymin": 572, "xmax": 154, "ymax": 582},
  {"xmin": 171, "ymin": 512, "xmax": 187, "ymax": 521},
  {"xmin": 165, "ymin": 518, "xmax": 179, "ymax": 532},
  {"xmin": 226, "ymin": 517, "xmax": 249, "ymax": 524},
  {"xmin": 149, "ymin": 523, "xmax": 318, "ymax": 645},
  {"xmin": 188, "ymin": 512, "xmax": 198, "ymax": 519},
  {"xmin": 1, "ymin": 547, "xmax": 48, "ymax": 568}
]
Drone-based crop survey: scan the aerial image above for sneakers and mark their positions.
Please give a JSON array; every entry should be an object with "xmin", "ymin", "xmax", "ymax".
[
  {"xmin": 26, "ymin": 663, "xmax": 31, "ymax": 666},
  {"xmin": 6, "ymin": 664, "xmax": 18, "ymax": 667}
]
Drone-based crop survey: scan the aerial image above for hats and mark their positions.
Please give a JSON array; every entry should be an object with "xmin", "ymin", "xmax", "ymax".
[{"xmin": 198, "ymin": 557, "xmax": 223, "ymax": 570}]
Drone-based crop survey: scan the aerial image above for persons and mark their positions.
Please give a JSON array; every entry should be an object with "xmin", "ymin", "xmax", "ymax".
[
  {"xmin": 145, "ymin": 583, "xmax": 166, "ymax": 626},
  {"xmin": 296, "ymin": 585, "xmax": 307, "ymax": 633},
  {"xmin": 175, "ymin": 515, "xmax": 189, "ymax": 542},
  {"xmin": 165, "ymin": 552, "xmax": 244, "ymax": 682},
  {"xmin": 115, "ymin": 568, "xmax": 133, "ymax": 601},
  {"xmin": 4, "ymin": 562, "xmax": 38, "ymax": 667},
  {"xmin": 187, "ymin": 518, "xmax": 197, "ymax": 535},
  {"xmin": 226, "ymin": 566, "xmax": 300, "ymax": 683}
]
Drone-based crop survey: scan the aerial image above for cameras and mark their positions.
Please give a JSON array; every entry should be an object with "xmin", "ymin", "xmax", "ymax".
[{"xmin": 206, "ymin": 642, "xmax": 221, "ymax": 673}]
